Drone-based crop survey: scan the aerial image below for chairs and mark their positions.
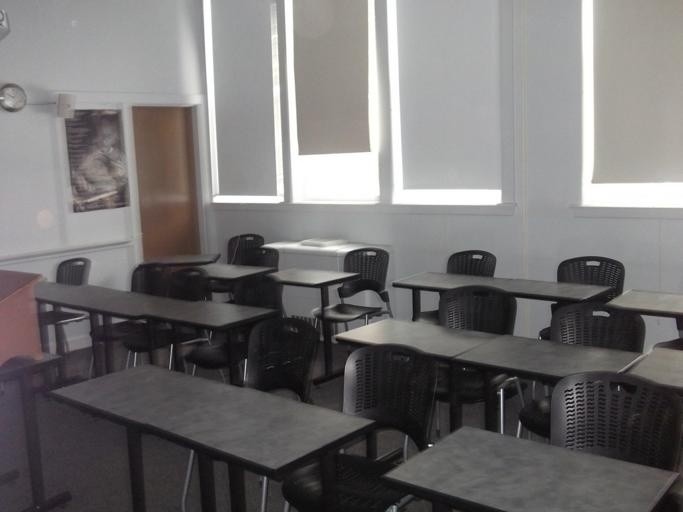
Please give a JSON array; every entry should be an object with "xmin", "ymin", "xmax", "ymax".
[
  {"xmin": 87, "ymin": 260, "xmax": 169, "ymax": 379},
  {"xmin": 281, "ymin": 338, "xmax": 437, "ymax": 511},
  {"xmin": 183, "ymin": 273, "xmax": 287, "ymax": 386},
  {"xmin": 420, "ymin": 250, "xmax": 497, "ymax": 321},
  {"xmin": 310, "ymin": 246, "xmax": 396, "ymax": 333},
  {"xmin": 227, "ymin": 234, "xmax": 264, "ymax": 265},
  {"xmin": 122, "ymin": 267, "xmax": 212, "ymax": 377},
  {"xmin": 550, "ymin": 369, "xmax": 680, "ymax": 481},
  {"xmin": 38, "ymin": 255, "xmax": 91, "ymax": 329},
  {"xmin": 179, "ymin": 313, "xmax": 329, "ymax": 511},
  {"xmin": 532, "ymin": 254, "xmax": 627, "ymax": 399},
  {"xmin": 512, "ymin": 300, "xmax": 646, "ymax": 444},
  {"xmin": 225, "ymin": 246, "xmax": 279, "ymax": 306},
  {"xmin": 402, "ymin": 285, "xmax": 525, "ymax": 462}
]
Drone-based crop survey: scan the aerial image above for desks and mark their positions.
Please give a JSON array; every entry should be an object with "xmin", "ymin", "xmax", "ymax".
[
  {"xmin": 201, "ymin": 261, "xmax": 276, "ymax": 301},
  {"xmin": 602, "ymin": 289, "xmax": 681, "ymax": 320},
  {"xmin": 1, "ymin": 350, "xmax": 73, "ymax": 510},
  {"xmin": 139, "ymin": 252, "xmax": 221, "ymax": 269},
  {"xmin": 337, "ymin": 318, "xmax": 502, "ymax": 437},
  {"xmin": 33, "ymin": 276, "xmax": 193, "ymax": 379},
  {"xmin": 452, "ymin": 334, "xmax": 645, "ymax": 437},
  {"xmin": 393, "ymin": 270, "xmax": 613, "ymax": 332},
  {"xmin": 145, "ymin": 289, "xmax": 283, "ymax": 380},
  {"xmin": 380, "ymin": 424, "xmax": 680, "ymax": 511},
  {"xmin": 268, "ymin": 265, "xmax": 362, "ymax": 386},
  {"xmin": 621, "ymin": 347, "xmax": 681, "ymax": 390},
  {"xmin": 261, "ymin": 239, "xmax": 391, "ymax": 270},
  {"xmin": 46, "ymin": 363, "xmax": 376, "ymax": 509}
]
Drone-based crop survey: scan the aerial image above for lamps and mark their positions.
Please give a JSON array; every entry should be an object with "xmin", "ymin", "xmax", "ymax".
[{"xmin": 54, "ymin": 92, "xmax": 77, "ymax": 120}]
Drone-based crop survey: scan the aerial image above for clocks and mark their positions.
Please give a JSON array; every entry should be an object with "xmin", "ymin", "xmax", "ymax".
[{"xmin": 0, "ymin": 83, "xmax": 27, "ymax": 112}]
